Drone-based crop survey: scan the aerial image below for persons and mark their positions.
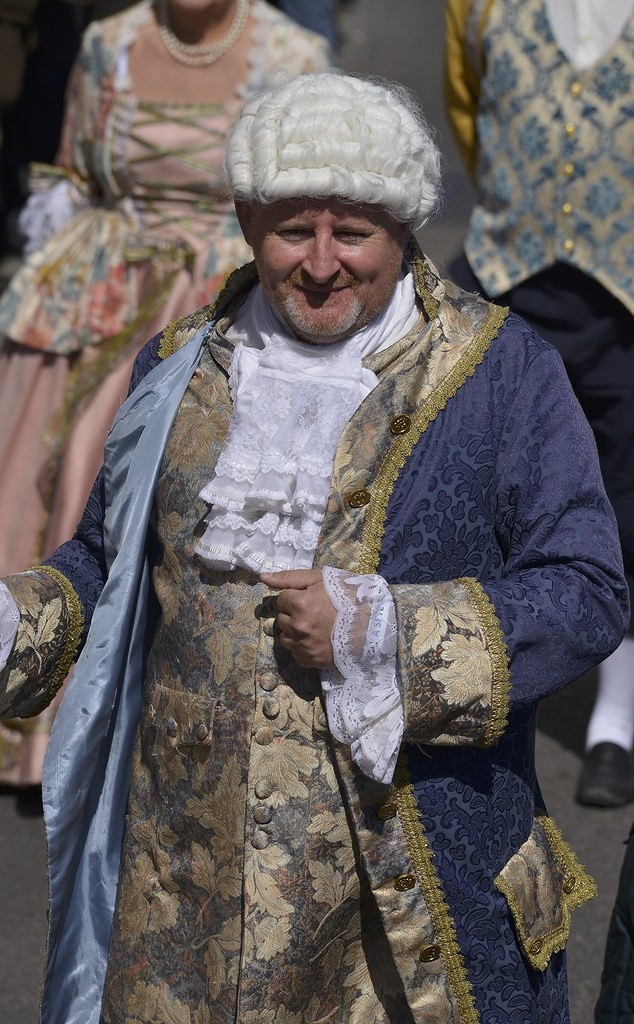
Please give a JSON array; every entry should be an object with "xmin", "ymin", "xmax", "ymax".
[
  {"xmin": 0, "ymin": 0, "xmax": 332, "ymax": 816},
  {"xmin": 439, "ymin": 1, "xmax": 634, "ymax": 807},
  {"xmin": 0, "ymin": 71, "xmax": 634, "ymax": 1024}
]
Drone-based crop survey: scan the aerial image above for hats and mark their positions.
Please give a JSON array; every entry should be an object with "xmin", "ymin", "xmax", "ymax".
[{"xmin": 224, "ymin": 68, "xmax": 444, "ymax": 225}]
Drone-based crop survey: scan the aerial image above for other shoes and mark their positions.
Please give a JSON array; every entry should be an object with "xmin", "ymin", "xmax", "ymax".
[
  {"xmin": 16, "ymin": 786, "xmax": 43, "ymax": 816},
  {"xmin": 574, "ymin": 743, "xmax": 634, "ymax": 806}
]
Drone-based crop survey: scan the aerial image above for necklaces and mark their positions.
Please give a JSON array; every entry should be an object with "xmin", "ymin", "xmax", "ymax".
[{"xmin": 157, "ymin": 0, "xmax": 250, "ymax": 66}]
